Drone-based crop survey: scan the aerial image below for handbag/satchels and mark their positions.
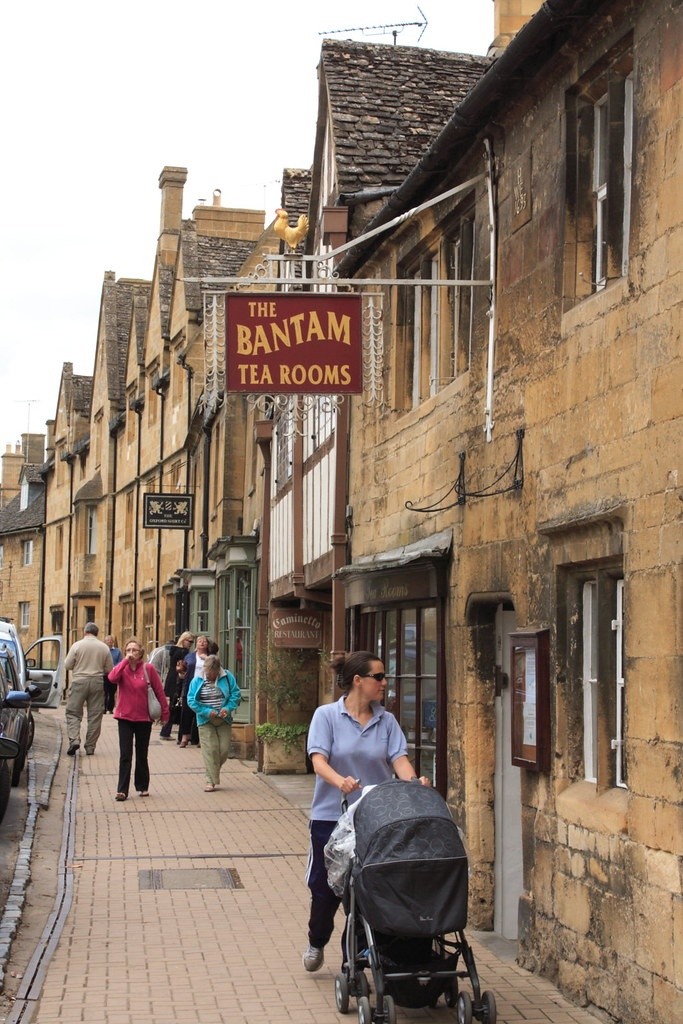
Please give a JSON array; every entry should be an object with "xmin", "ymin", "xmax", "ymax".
[{"xmin": 143, "ymin": 663, "xmax": 162, "ymax": 721}]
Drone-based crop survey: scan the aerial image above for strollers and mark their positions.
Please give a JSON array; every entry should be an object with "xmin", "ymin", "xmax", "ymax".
[{"xmin": 335, "ymin": 776, "xmax": 497, "ymax": 1024}]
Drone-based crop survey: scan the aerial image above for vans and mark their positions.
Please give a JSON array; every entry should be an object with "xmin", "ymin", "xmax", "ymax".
[{"xmin": 0, "ymin": 617, "xmax": 62, "ymax": 749}]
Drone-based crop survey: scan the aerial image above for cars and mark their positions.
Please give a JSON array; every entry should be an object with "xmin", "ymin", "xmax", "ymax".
[{"xmin": 0, "ymin": 663, "xmax": 32, "ymax": 825}]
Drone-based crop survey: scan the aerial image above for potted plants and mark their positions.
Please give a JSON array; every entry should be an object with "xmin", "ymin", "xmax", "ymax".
[{"xmin": 250, "ymin": 626, "xmax": 331, "ymax": 769}]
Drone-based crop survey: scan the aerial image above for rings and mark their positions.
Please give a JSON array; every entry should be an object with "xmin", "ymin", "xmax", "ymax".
[{"xmin": 224, "ymin": 713, "xmax": 225, "ymax": 715}]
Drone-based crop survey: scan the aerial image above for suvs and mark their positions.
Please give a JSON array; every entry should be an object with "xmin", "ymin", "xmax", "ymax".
[{"xmin": 1, "ymin": 642, "xmax": 41, "ymax": 787}]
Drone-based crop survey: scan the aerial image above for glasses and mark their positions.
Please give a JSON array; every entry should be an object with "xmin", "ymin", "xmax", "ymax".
[
  {"xmin": 360, "ymin": 673, "xmax": 386, "ymax": 681},
  {"xmin": 187, "ymin": 640, "xmax": 194, "ymax": 643},
  {"xmin": 125, "ymin": 649, "xmax": 141, "ymax": 653}
]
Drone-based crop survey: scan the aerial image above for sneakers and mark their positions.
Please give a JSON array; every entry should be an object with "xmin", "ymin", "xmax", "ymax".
[{"xmin": 303, "ymin": 943, "xmax": 324, "ymax": 972}]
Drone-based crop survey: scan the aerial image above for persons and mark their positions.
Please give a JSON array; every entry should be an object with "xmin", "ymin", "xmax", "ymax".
[
  {"xmin": 108, "ymin": 640, "xmax": 170, "ymax": 801},
  {"xmin": 64, "ymin": 621, "xmax": 123, "ymax": 755},
  {"xmin": 304, "ymin": 650, "xmax": 432, "ymax": 972},
  {"xmin": 148, "ymin": 631, "xmax": 242, "ymax": 791}
]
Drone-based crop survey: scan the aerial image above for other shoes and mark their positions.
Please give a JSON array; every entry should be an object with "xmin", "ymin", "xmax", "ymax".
[
  {"xmin": 179, "ymin": 735, "xmax": 190, "ymax": 747},
  {"xmin": 139, "ymin": 791, "xmax": 149, "ymax": 796},
  {"xmin": 67, "ymin": 740, "xmax": 80, "ymax": 755},
  {"xmin": 86, "ymin": 747, "xmax": 94, "ymax": 755},
  {"xmin": 205, "ymin": 782, "xmax": 215, "ymax": 792},
  {"xmin": 160, "ymin": 735, "xmax": 176, "ymax": 740},
  {"xmin": 115, "ymin": 792, "xmax": 126, "ymax": 801},
  {"xmin": 105, "ymin": 710, "xmax": 113, "ymax": 714}
]
